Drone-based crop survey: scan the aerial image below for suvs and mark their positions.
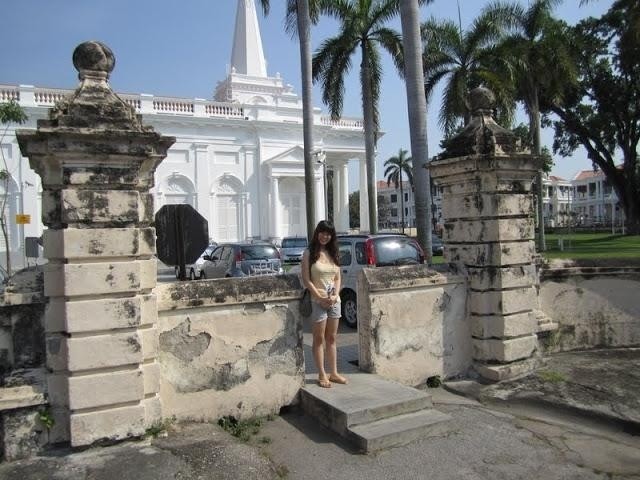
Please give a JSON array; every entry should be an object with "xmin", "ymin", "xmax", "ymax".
[
  {"xmin": 200, "ymin": 239, "xmax": 282, "ymax": 280},
  {"xmin": 288, "ymin": 232, "xmax": 425, "ymax": 330}
]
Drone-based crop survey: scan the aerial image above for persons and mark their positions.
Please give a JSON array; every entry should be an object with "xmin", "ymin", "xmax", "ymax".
[{"xmin": 301, "ymin": 220, "xmax": 348, "ymax": 387}]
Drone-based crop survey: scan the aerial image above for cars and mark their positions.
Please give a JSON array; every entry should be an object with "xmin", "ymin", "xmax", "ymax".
[
  {"xmin": 431, "ymin": 232, "xmax": 444, "ymax": 255},
  {"xmin": 279, "ymin": 236, "xmax": 308, "ymax": 263},
  {"xmin": 175, "ymin": 237, "xmax": 219, "ymax": 280}
]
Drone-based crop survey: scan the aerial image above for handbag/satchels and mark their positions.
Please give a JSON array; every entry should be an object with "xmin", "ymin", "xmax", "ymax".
[{"xmin": 299, "ymin": 288, "xmax": 313, "ymax": 317}]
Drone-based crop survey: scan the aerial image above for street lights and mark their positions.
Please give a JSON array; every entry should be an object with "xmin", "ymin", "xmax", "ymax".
[{"xmin": 318, "ymin": 160, "xmax": 329, "ymax": 223}]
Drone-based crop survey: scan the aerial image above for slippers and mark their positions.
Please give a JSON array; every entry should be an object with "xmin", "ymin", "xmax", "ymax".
[{"xmin": 318, "ymin": 374, "xmax": 349, "ymax": 388}]
